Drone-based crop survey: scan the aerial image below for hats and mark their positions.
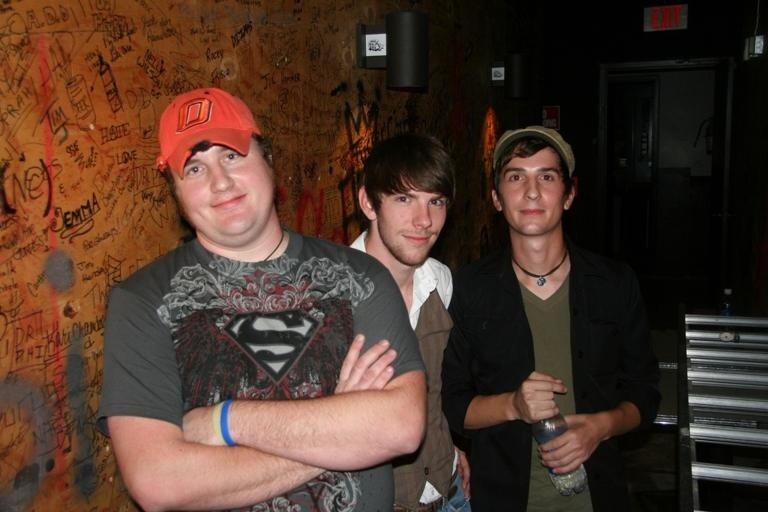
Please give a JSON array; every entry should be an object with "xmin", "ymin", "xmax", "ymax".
[
  {"xmin": 154, "ymin": 87, "xmax": 262, "ymax": 180},
  {"xmin": 493, "ymin": 125, "xmax": 577, "ymax": 180}
]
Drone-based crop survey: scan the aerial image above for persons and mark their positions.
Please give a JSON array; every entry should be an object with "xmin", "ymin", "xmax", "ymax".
[
  {"xmin": 346, "ymin": 133, "xmax": 472, "ymax": 512},
  {"xmin": 95, "ymin": 87, "xmax": 428, "ymax": 512},
  {"xmin": 441, "ymin": 125, "xmax": 664, "ymax": 512}
]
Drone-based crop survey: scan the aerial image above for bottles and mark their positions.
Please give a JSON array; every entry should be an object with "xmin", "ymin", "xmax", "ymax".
[
  {"xmin": 533, "ymin": 415, "xmax": 589, "ymax": 497},
  {"xmin": 718, "ymin": 288, "xmax": 736, "ymax": 316}
]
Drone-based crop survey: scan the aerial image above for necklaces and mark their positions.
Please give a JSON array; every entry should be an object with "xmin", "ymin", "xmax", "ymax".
[
  {"xmin": 512, "ymin": 250, "xmax": 566, "ymax": 286},
  {"xmin": 264, "ymin": 234, "xmax": 284, "ymax": 261}
]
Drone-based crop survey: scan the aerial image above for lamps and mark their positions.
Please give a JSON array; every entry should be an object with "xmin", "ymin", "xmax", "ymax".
[{"xmin": 354, "ymin": 8, "xmax": 436, "ymax": 91}]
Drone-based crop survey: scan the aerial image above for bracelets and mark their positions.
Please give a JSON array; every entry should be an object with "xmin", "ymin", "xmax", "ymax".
[
  {"xmin": 221, "ymin": 399, "xmax": 235, "ymax": 446},
  {"xmin": 213, "ymin": 401, "xmax": 227, "ymax": 446}
]
